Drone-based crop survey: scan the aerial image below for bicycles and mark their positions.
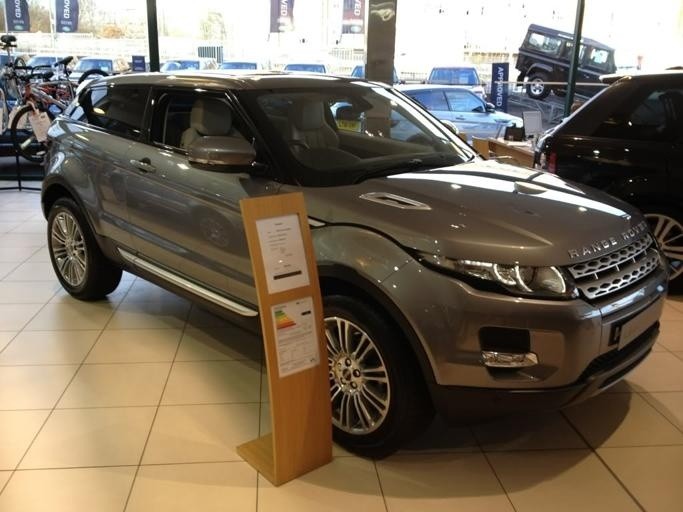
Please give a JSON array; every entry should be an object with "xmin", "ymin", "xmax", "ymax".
[{"xmin": 0, "ymin": 35, "xmax": 109, "ymax": 163}]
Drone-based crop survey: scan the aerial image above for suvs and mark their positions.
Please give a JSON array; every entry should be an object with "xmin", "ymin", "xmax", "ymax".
[
  {"xmin": 39, "ymin": 70, "xmax": 671, "ymax": 462},
  {"xmin": 534, "ymin": 70, "xmax": 682, "ymax": 297},
  {"xmin": 514, "ymin": 23, "xmax": 617, "ymax": 101}
]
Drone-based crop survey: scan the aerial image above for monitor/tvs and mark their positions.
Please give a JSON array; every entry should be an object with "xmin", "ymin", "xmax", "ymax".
[{"xmin": 522, "ymin": 111, "xmax": 542, "ymax": 136}]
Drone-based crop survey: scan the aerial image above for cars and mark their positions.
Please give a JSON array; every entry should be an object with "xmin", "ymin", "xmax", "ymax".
[
  {"xmin": 160, "ymin": 59, "xmax": 204, "ymax": 70},
  {"xmin": 350, "ymin": 64, "xmax": 405, "ymax": 85},
  {"xmin": 329, "ymin": 83, "xmax": 526, "ymax": 147},
  {"xmin": 420, "ymin": 66, "xmax": 487, "ymax": 101},
  {"xmin": 282, "ymin": 63, "xmax": 328, "ymax": 74},
  {"xmin": 216, "ymin": 61, "xmax": 263, "ymax": 69}
]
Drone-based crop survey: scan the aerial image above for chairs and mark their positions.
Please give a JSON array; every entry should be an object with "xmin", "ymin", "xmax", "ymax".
[{"xmin": 104, "ymin": 85, "xmax": 341, "ymax": 165}]
